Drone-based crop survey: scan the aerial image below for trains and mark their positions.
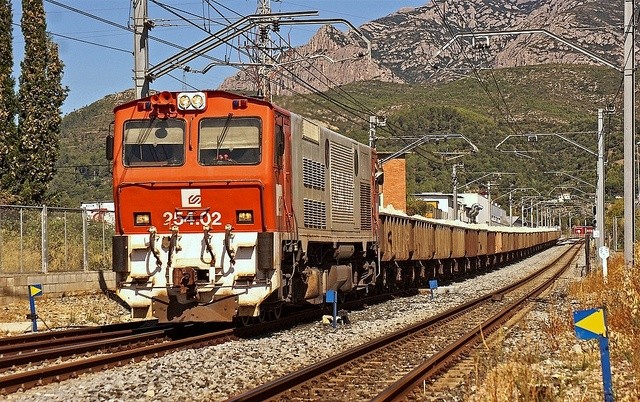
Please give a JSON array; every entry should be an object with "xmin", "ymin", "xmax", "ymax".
[{"xmin": 106, "ymin": 91, "xmax": 561, "ymax": 326}]
